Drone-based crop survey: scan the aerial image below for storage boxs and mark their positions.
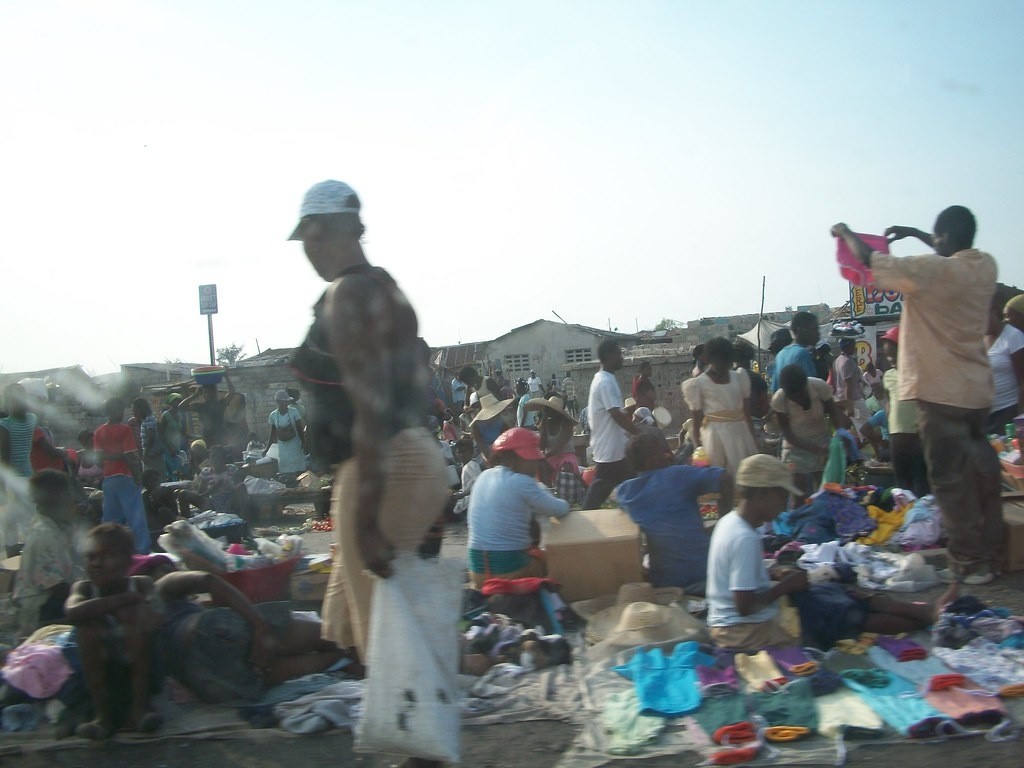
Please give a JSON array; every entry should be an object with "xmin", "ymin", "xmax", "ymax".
[
  {"xmin": 541, "ymin": 510, "xmax": 642, "ymax": 602},
  {"xmin": 999, "ymin": 490, "xmax": 1024, "ymax": 572}
]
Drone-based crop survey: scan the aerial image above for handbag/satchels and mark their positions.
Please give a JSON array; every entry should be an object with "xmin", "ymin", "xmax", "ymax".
[{"xmin": 277, "ymin": 408, "xmax": 296, "ymax": 441}]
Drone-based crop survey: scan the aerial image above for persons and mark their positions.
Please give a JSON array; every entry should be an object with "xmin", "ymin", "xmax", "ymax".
[
  {"xmin": 770, "ymin": 364, "xmax": 847, "ymax": 509},
  {"xmin": 834, "ymin": 337, "xmax": 873, "ymax": 442},
  {"xmin": 289, "ymin": 179, "xmax": 449, "ymax": 666},
  {"xmin": 860, "ymin": 409, "xmax": 891, "ymax": 462},
  {"xmin": 0, "ymin": 362, "xmax": 659, "ymax": 555},
  {"xmin": 1002, "ymin": 293, "xmax": 1024, "ymax": 330},
  {"xmin": 66, "ymin": 521, "xmax": 162, "ymax": 736},
  {"xmin": 981, "ymin": 291, "xmax": 1024, "ymax": 438},
  {"xmin": 833, "ymin": 204, "xmax": 1012, "ymax": 586},
  {"xmin": 14, "ymin": 468, "xmax": 86, "ymax": 624},
  {"xmin": 131, "ymin": 556, "xmax": 285, "ymax": 702},
  {"xmin": 179, "ymin": 444, "xmax": 249, "ymax": 521},
  {"xmin": 707, "ymin": 455, "xmax": 960, "ymax": 647},
  {"xmin": 615, "ymin": 432, "xmax": 727, "ymax": 594},
  {"xmin": 583, "ymin": 339, "xmax": 642, "ymax": 511},
  {"xmin": 466, "ymin": 428, "xmax": 570, "ymax": 592},
  {"xmin": 678, "ymin": 311, "xmax": 830, "ymax": 468},
  {"xmin": 880, "ymin": 326, "xmax": 930, "ymax": 499},
  {"xmin": 863, "ymin": 362, "xmax": 883, "ymax": 385},
  {"xmin": 94, "ymin": 397, "xmax": 153, "ymax": 556}
]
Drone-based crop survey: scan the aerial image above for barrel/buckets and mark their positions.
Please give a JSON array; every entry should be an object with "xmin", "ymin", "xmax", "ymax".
[
  {"xmin": 199, "ymin": 522, "xmax": 247, "ymax": 549},
  {"xmin": 213, "ymin": 551, "xmax": 306, "ymax": 604},
  {"xmin": 652, "ymin": 406, "xmax": 673, "ymax": 430}
]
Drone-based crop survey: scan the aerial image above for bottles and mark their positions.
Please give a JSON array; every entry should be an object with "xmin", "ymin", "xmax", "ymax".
[{"xmin": 986, "ymin": 421, "xmax": 1023, "ymax": 465}]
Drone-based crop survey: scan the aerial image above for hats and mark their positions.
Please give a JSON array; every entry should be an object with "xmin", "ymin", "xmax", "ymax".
[
  {"xmin": 622, "ymin": 398, "xmax": 638, "ymax": 409},
  {"xmin": 572, "ymin": 578, "xmax": 708, "ymax": 653},
  {"xmin": 275, "ymin": 390, "xmax": 294, "ymax": 402},
  {"xmin": 492, "ymin": 426, "xmax": 545, "ymax": 460},
  {"xmin": 816, "ymin": 341, "xmax": 832, "ymax": 352},
  {"xmin": 880, "ymin": 326, "xmax": 899, "ymax": 344},
  {"xmin": 288, "ymin": 179, "xmax": 361, "ymax": 241},
  {"xmin": 468, "ymin": 393, "xmax": 514, "ymax": 427},
  {"xmin": 525, "ymin": 396, "xmax": 580, "ymax": 426},
  {"xmin": 737, "ymin": 454, "xmax": 804, "ymax": 496}
]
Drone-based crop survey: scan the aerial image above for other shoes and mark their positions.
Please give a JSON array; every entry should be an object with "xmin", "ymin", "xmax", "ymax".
[
  {"xmin": 75, "ymin": 721, "xmax": 114, "ymax": 739},
  {"xmin": 937, "ymin": 567, "xmax": 994, "ymax": 585},
  {"xmin": 137, "ymin": 712, "xmax": 161, "ymax": 731}
]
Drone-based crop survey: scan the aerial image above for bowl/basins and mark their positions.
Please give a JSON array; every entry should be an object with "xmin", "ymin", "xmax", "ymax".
[{"xmin": 191, "ymin": 365, "xmax": 226, "ymax": 386}]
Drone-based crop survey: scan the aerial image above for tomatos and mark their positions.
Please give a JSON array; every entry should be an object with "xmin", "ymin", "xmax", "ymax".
[{"xmin": 312, "ymin": 517, "xmax": 333, "ymax": 531}]
